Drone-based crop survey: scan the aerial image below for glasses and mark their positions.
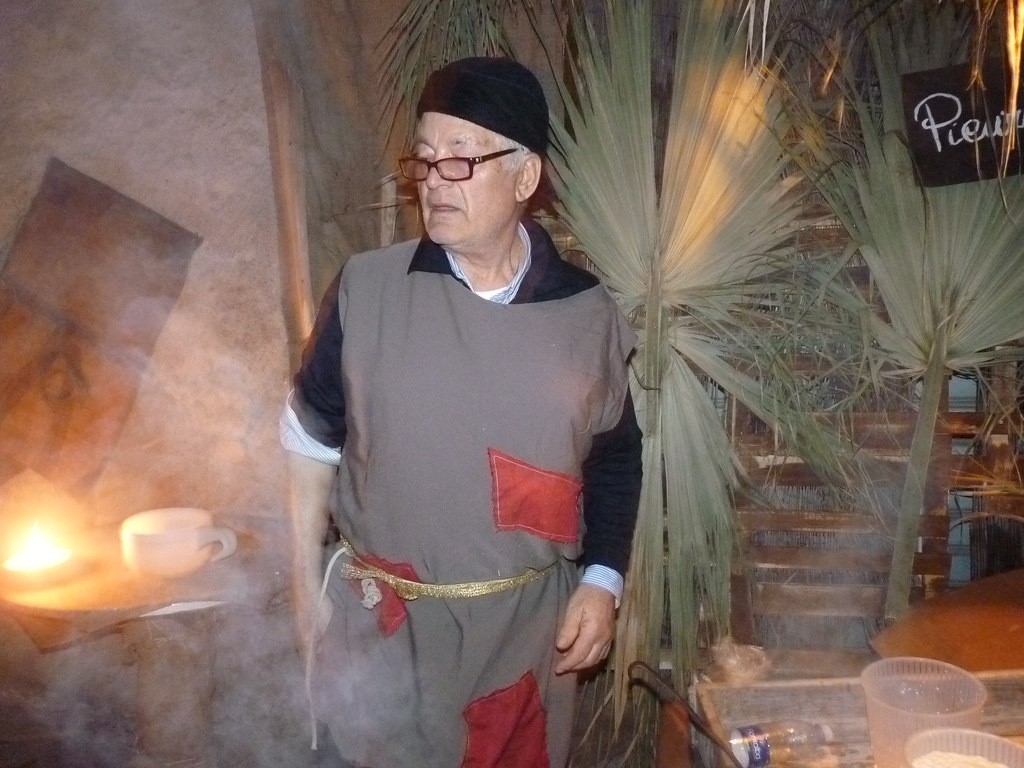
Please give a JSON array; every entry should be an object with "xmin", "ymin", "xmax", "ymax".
[{"xmin": 398, "ymin": 148, "xmax": 526, "ymax": 182}]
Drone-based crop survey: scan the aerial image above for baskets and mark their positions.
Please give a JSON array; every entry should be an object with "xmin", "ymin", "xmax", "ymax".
[
  {"xmin": 861, "ymin": 654, "xmax": 988, "ymax": 768},
  {"xmin": 903, "ymin": 725, "xmax": 1024, "ymax": 768}
]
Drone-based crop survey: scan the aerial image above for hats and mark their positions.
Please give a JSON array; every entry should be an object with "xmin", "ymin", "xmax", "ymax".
[{"xmin": 416, "ymin": 57, "xmax": 549, "ymax": 158}]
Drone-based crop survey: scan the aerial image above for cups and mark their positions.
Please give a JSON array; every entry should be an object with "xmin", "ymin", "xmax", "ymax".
[
  {"xmin": 119, "ymin": 506, "xmax": 237, "ymax": 578},
  {"xmin": 902, "ymin": 727, "xmax": 1024, "ymax": 768},
  {"xmin": 861, "ymin": 655, "xmax": 987, "ymax": 768}
]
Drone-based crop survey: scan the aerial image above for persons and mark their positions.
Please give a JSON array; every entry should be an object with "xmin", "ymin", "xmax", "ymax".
[{"xmin": 280, "ymin": 59, "xmax": 643, "ymax": 768}]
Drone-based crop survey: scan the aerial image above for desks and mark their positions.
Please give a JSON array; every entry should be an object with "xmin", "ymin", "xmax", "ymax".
[{"xmin": 686, "ymin": 668, "xmax": 1024, "ymax": 768}]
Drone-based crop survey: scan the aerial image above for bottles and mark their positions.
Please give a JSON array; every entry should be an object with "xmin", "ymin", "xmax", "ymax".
[{"xmin": 725, "ymin": 720, "xmax": 832, "ymax": 768}]
